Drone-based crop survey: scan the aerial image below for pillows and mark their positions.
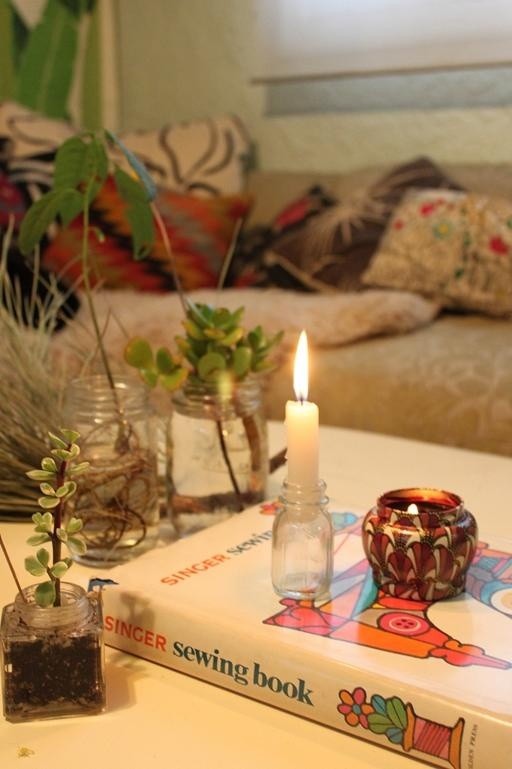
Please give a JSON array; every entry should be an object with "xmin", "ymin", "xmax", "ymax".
[
  {"xmin": 1, "ymin": 140, "xmax": 82, "ymax": 280},
  {"xmin": 40, "ymin": 174, "xmax": 253, "ymax": 294},
  {"xmin": 370, "ymin": 183, "xmax": 511, "ymax": 306},
  {"xmin": 270, "ymin": 157, "xmax": 465, "ymax": 295}
]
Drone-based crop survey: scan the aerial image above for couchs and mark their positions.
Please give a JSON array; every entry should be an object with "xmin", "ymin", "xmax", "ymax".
[{"xmin": 1, "ymin": 162, "xmax": 512, "ymax": 461}]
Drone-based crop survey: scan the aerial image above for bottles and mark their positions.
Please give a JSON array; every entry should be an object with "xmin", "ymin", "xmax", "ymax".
[
  {"xmin": 265, "ymin": 479, "xmax": 334, "ymax": 598},
  {"xmin": 0, "ymin": 579, "xmax": 109, "ymax": 728},
  {"xmin": 46, "ymin": 368, "xmax": 158, "ymax": 574},
  {"xmin": 156, "ymin": 371, "xmax": 275, "ymax": 542}
]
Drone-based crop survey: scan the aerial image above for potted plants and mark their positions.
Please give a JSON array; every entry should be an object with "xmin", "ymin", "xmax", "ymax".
[
  {"xmin": 0, "ymin": 425, "xmax": 114, "ymax": 720},
  {"xmin": 17, "ymin": 133, "xmax": 162, "ymax": 569},
  {"xmin": 127, "ymin": 291, "xmax": 282, "ymax": 533}
]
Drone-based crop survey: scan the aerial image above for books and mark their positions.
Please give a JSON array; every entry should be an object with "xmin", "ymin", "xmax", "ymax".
[{"xmin": 91, "ymin": 495, "xmax": 512, "ymax": 769}]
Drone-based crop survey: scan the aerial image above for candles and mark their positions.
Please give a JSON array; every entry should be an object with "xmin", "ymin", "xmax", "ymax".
[{"xmin": 285, "ymin": 330, "xmax": 320, "ymax": 522}]
